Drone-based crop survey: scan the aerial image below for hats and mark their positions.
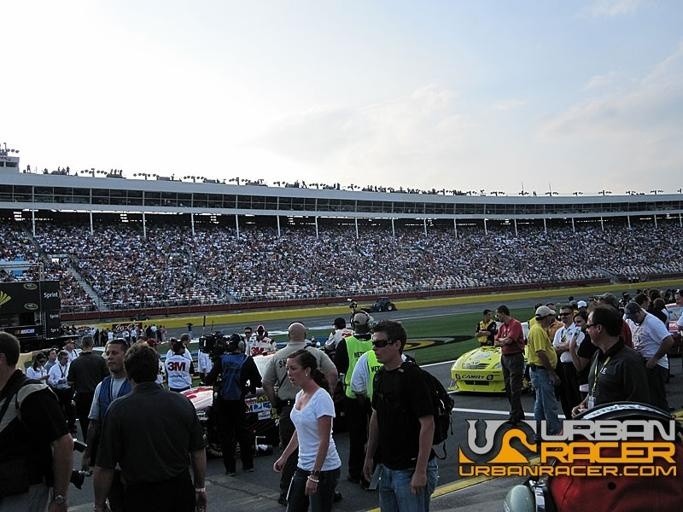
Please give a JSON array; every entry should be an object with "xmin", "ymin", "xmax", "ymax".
[
  {"xmin": 354, "ymin": 313, "xmax": 373, "ymax": 326},
  {"xmin": 577, "ymin": 301, "xmax": 587, "ymax": 308},
  {"xmin": 593, "ymin": 293, "xmax": 619, "ymax": 307},
  {"xmin": 535, "ymin": 306, "xmax": 556, "ymax": 317}
]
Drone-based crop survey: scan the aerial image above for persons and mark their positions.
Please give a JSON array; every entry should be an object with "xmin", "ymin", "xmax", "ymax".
[{"xmin": 0, "ymin": 223, "xmax": 683, "ymax": 510}]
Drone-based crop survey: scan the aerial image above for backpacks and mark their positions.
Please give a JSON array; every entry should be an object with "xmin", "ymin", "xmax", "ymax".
[{"xmin": 397, "ymin": 362, "xmax": 450, "ymax": 445}]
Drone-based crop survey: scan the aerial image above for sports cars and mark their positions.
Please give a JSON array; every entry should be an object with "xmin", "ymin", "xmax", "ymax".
[
  {"xmin": 178, "ymin": 353, "xmax": 352, "ymax": 457},
  {"xmin": 448, "ymin": 321, "xmax": 539, "ymax": 394},
  {"xmin": 665, "ymin": 303, "xmax": 682, "ymax": 354}
]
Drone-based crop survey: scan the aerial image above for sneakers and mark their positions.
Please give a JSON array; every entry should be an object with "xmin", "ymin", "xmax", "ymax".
[
  {"xmin": 347, "ymin": 472, "xmax": 376, "ymax": 492},
  {"xmin": 226, "ymin": 468, "xmax": 255, "ymax": 476}
]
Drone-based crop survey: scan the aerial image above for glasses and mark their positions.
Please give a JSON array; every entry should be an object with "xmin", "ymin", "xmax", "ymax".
[
  {"xmin": 559, "ymin": 313, "xmax": 571, "ymax": 316},
  {"xmin": 371, "ymin": 341, "xmax": 392, "ymax": 348}
]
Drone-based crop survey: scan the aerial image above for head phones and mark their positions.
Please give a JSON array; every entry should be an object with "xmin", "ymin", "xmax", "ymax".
[
  {"xmin": 230, "ymin": 331, "xmax": 244, "ymax": 353},
  {"xmin": 348, "ymin": 310, "xmax": 373, "ymax": 331}
]
